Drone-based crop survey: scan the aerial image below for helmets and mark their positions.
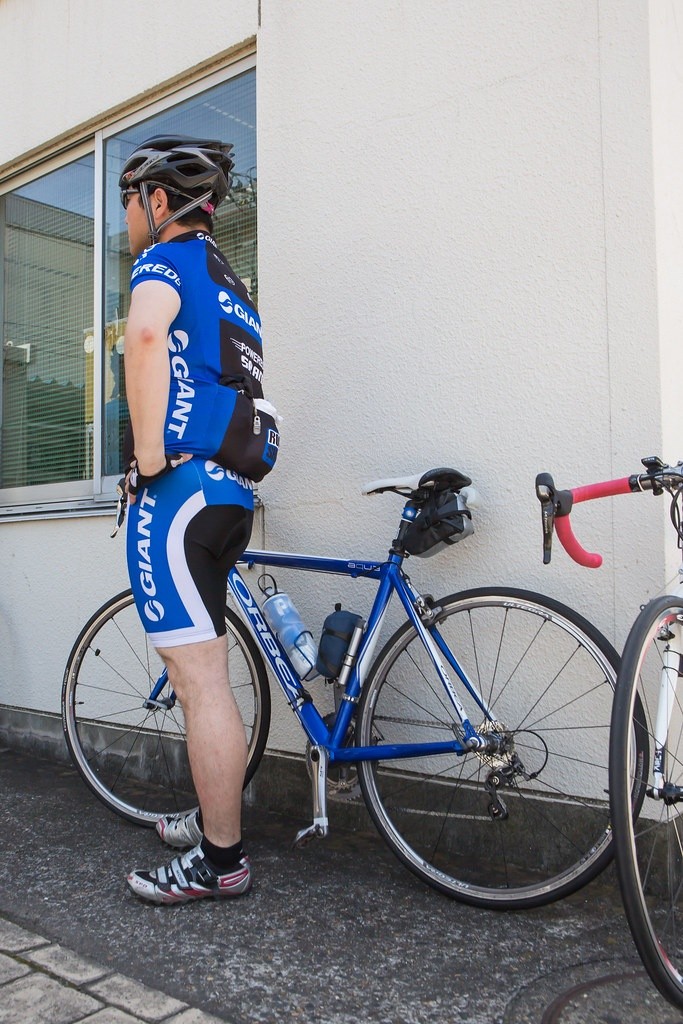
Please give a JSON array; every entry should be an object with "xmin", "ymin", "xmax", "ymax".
[{"xmin": 116, "ymin": 134, "xmax": 236, "ymax": 202}]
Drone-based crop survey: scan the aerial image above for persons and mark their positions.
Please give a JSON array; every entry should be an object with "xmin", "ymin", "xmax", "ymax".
[{"xmin": 118, "ymin": 134, "xmax": 279, "ymax": 906}]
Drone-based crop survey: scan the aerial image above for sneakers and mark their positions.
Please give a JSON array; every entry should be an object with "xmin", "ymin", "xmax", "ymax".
[
  {"xmin": 124, "ymin": 836, "xmax": 254, "ymax": 905},
  {"xmin": 155, "ymin": 810, "xmax": 204, "ymax": 852}
]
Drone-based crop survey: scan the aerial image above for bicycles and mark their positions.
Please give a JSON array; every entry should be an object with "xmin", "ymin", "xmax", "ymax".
[
  {"xmin": 56, "ymin": 465, "xmax": 650, "ymax": 909},
  {"xmin": 533, "ymin": 456, "xmax": 683, "ymax": 1008}
]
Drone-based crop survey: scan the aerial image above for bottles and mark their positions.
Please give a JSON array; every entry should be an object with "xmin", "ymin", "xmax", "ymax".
[{"xmin": 260, "ymin": 586, "xmax": 318, "ymax": 681}]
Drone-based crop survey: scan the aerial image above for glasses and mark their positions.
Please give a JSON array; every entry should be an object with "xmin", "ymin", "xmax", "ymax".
[{"xmin": 120, "ymin": 189, "xmax": 139, "ymax": 209}]
